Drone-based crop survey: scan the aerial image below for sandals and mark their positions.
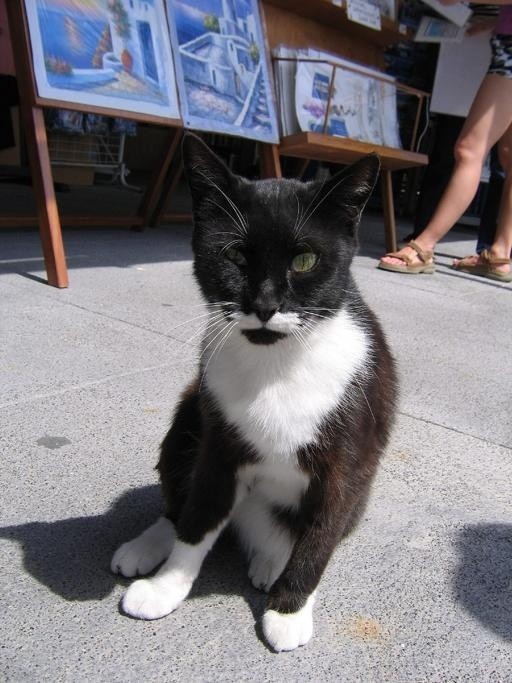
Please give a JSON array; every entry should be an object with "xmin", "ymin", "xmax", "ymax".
[
  {"xmin": 379, "ymin": 239, "xmax": 436, "ymax": 275},
  {"xmin": 451, "ymin": 249, "xmax": 512, "ymax": 283}
]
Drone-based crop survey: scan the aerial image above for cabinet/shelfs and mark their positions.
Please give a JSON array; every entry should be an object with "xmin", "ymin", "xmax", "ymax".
[{"xmin": 269, "ymin": 44, "xmax": 431, "ymax": 259}]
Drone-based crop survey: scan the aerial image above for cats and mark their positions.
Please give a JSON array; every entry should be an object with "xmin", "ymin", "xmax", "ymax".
[{"xmin": 104, "ymin": 129, "xmax": 401, "ymax": 655}]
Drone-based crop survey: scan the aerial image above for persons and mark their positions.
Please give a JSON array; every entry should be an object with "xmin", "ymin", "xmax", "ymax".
[{"xmin": 376, "ymin": 0, "xmax": 511, "ymax": 282}]
